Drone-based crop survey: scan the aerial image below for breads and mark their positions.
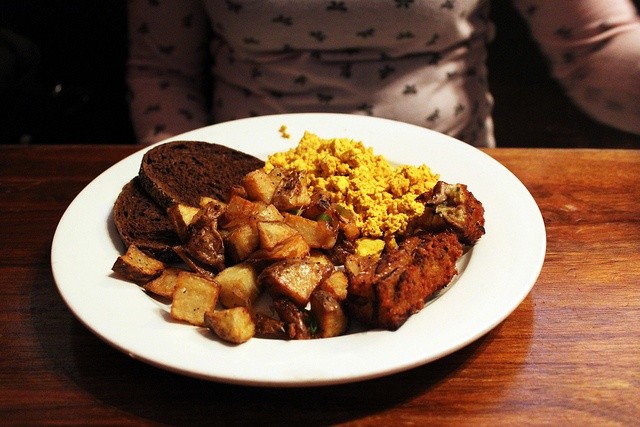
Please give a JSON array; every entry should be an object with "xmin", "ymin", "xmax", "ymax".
[
  {"xmin": 107, "ymin": 176, "xmax": 182, "ymax": 268},
  {"xmin": 140, "ymin": 141, "xmax": 284, "ymax": 209}
]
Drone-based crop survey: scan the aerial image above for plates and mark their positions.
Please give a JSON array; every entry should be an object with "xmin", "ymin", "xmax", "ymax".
[{"xmin": 51, "ymin": 112, "xmax": 548, "ymax": 389}]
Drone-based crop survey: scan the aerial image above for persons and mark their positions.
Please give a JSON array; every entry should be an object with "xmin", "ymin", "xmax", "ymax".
[{"xmin": 125, "ymin": 0, "xmax": 640, "ymax": 144}]
